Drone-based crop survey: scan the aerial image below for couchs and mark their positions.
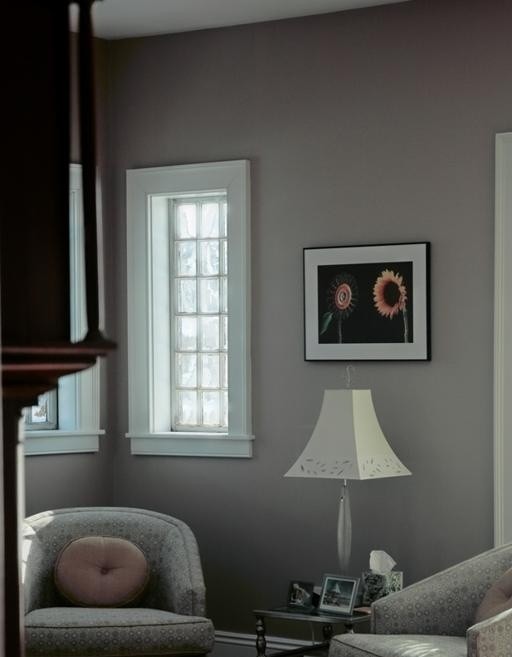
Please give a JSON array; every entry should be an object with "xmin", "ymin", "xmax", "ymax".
[
  {"xmin": 19, "ymin": 508, "xmax": 215, "ymax": 657},
  {"xmin": 330, "ymin": 545, "xmax": 511, "ymax": 655}
]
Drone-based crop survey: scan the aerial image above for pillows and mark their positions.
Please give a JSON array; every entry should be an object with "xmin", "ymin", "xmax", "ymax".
[
  {"xmin": 474, "ymin": 567, "xmax": 511, "ymax": 622},
  {"xmin": 57, "ymin": 535, "xmax": 149, "ymax": 606}
]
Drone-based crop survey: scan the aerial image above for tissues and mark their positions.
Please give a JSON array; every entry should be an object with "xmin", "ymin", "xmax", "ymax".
[{"xmin": 361, "ymin": 550, "xmax": 402, "ymax": 605}]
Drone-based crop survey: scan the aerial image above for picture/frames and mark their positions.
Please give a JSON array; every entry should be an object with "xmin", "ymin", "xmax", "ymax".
[
  {"xmin": 319, "ymin": 572, "xmax": 361, "ymax": 617},
  {"xmin": 303, "ymin": 241, "xmax": 430, "ymax": 362},
  {"xmin": 287, "ymin": 580, "xmax": 317, "ymax": 615}
]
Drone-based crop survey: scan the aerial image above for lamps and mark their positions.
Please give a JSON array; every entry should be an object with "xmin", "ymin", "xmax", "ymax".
[{"xmin": 285, "ymin": 366, "xmax": 411, "ymax": 565}]
